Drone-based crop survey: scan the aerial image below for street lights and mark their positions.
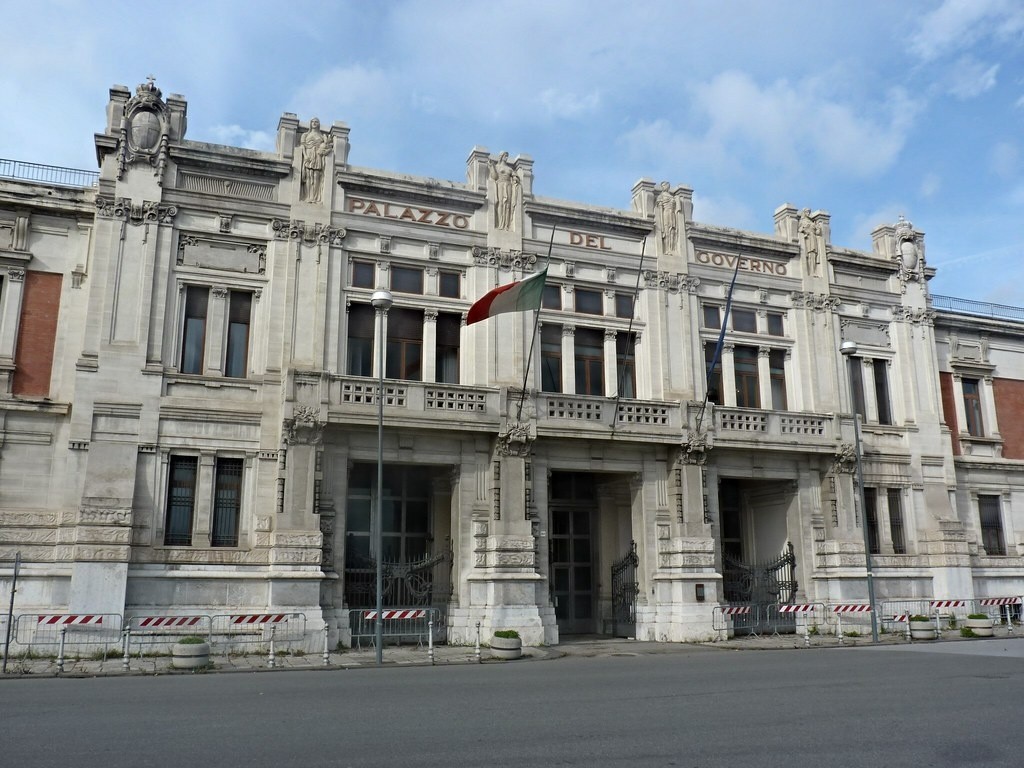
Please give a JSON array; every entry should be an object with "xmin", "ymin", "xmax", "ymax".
[
  {"xmin": 839, "ymin": 339, "xmax": 881, "ymax": 645},
  {"xmin": 372, "ymin": 286, "xmax": 391, "ymax": 663}
]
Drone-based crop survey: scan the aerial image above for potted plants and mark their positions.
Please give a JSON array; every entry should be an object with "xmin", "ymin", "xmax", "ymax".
[
  {"xmin": 173, "ymin": 637, "xmax": 210, "ymax": 669},
  {"xmin": 965, "ymin": 613, "xmax": 993, "ymax": 637},
  {"xmin": 490, "ymin": 629, "xmax": 522, "ymax": 660},
  {"xmin": 908, "ymin": 614, "xmax": 937, "ymax": 640}
]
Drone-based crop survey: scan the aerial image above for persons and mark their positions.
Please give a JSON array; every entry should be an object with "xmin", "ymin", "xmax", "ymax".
[
  {"xmin": 487, "ymin": 151, "xmax": 519, "ymax": 231},
  {"xmin": 301, "ymin": 117, "xmax": 333, "ymax": 202},
  {"xmin": 798, "ymin": 208, "xmax": 821, "ymax": 274},
  {"xmin": 657, "ymin": 181, "xmax": 676, "ymax": 255}
]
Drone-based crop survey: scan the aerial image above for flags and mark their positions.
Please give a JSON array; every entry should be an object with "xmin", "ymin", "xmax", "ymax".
[
  {"xmin": 466, "ymin": 269, "xmax": 548, "ymax": 326},
  {"xmin": 707, "ymin": 265, "xmax": 737, "ymax": 373}
]
